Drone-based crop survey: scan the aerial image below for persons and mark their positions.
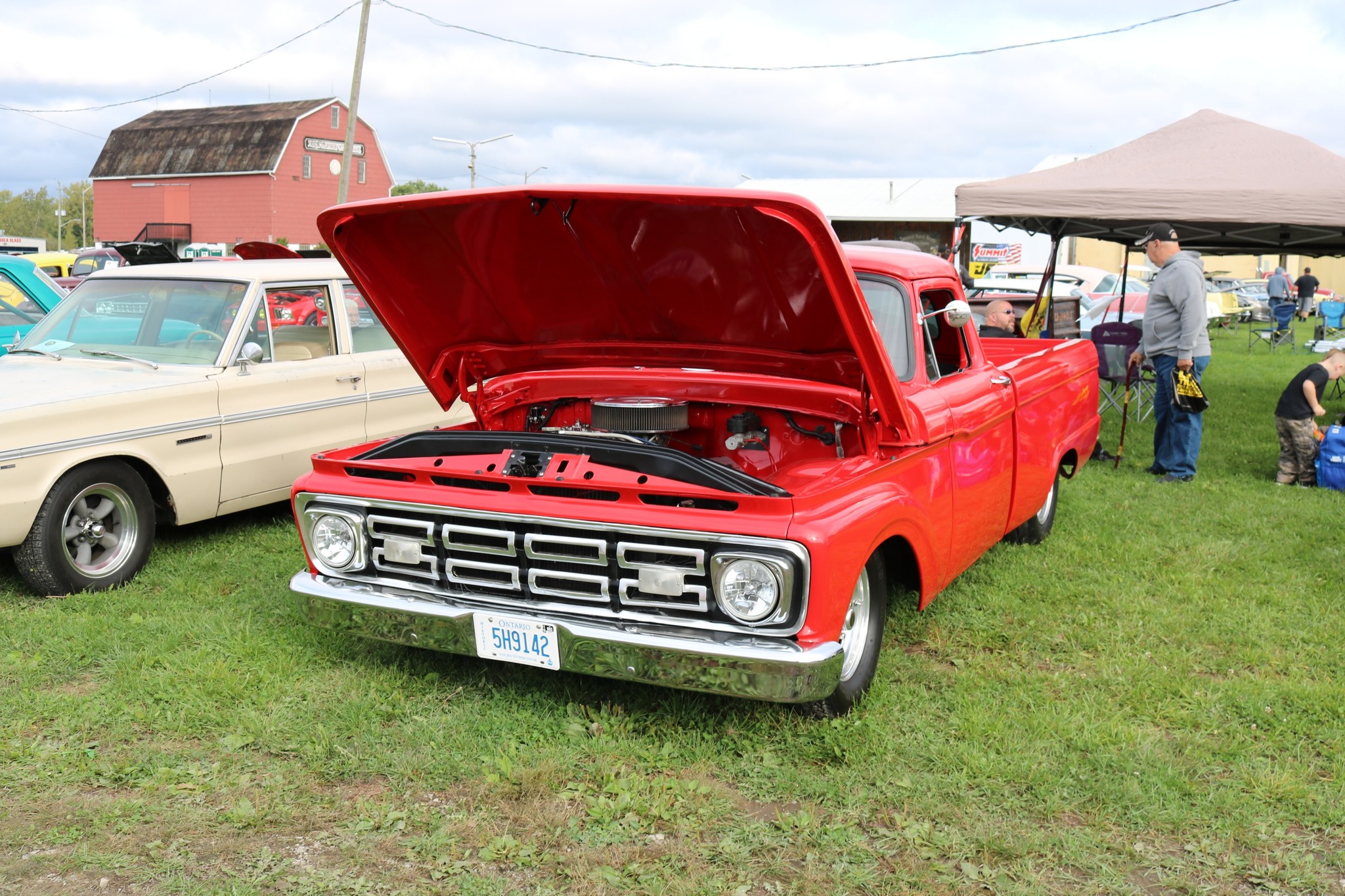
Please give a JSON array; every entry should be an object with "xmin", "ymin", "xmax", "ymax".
[
  {"xmin": 977, "ymin": 299, "xmax": 1118, "ymax": 462},
  {"xmin": 1291, "ymin": 267, "xmax": 1320, "ymax": 324},
  {"xmin": 1127, "ymin": 222, "xmax": 1211, "ymax": 485},
  {"xmin": 1273, "ymin": 348, "xmax": 1345, "ymax": 488},
  {"xmin": 1266, "ymin": 267, "xmax": 1292, "ymax": 323}
]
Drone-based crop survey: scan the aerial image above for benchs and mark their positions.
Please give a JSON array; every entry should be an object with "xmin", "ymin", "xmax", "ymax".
[{"xmin": 261, "ymin": 325, "xmax": 399, "ymax": 358}]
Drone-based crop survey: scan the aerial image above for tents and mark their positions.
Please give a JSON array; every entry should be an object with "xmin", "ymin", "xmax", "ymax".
[{"xmin": 951, "ymin": 108, "xmax": 1345, "ymax": 332}]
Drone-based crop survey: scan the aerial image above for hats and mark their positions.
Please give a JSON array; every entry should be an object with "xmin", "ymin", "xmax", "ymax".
[{"xmin": 1134, "ymin": 222, "xmax": 1178, "ymax": 246}]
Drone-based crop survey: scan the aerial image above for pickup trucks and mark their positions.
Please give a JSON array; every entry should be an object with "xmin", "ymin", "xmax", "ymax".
[
  {"xmin": 841, "ymin": 240, "xmax": 1083, "ymax": 341},
  {"xmin": 289, "ymin": 188, "xmax": 1101, "ymax": 718}
]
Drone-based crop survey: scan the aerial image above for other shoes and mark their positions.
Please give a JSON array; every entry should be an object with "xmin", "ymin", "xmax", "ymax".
[
  {"xmin": 1145, "ymin": 465, "xmax": 1167, "ymax": 475},
  {"xmin": 1299, "ymin": 481, "xmax": 1316, "ymax": 489},
  {"xmin": 1091, "ymin": 451, "xmax": 1123, "ymax": 460},
  {"xmin": 1275, "ymin": 481, "xmax": 1289, "ymax": 486},
  {"xmin": 1154, "ymin": 472, "xmax": 1194, "ymax": 485}
]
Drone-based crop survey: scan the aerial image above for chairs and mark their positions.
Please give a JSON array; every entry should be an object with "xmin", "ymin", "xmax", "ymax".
[
  {"xmin": 1207, "ymin": 293, "xmax": 1296, "ymax": 356},
  {"xmin": 263, "ymin": 342, "xmax": 328, "ymax": 362},
  {"xmin": 1091, "ymin": 319, "xmax": 1156, "ymax": 424},
  {"xmin": 1313, "ymin": 302, "xmax": 1345, "ymax": 341},
  {"xmin": 165, "ymin": 340, "xmax": 222, "ymax": 352}
]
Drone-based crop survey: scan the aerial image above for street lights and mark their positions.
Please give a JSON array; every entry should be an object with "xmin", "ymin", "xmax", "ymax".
[
  {"xmin": 432, "ymin": 134, "xmax": 513, "ymax": 189},
  {"xmin": 525, "ymin": 167, "xmax": 547, "ymax": 185},
  {"xmin": 58, "ymin": 219, "xmax": 81, "ymax": 253}
]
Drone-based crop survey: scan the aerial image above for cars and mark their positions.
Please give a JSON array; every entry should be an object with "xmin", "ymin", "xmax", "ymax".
[
  {"xmin": 0, "ymin": 239, "xmax": 488, "ymax": 599},
  {"xmin": 974, "ymin": 264, "xmax": 1335, "ymax": 334}
]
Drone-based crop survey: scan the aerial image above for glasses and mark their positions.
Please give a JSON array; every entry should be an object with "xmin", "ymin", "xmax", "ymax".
[
  {"xmin": 1142, "ymin": 240, "xmax": 1152, "ymax": 254},
  {"xmin": 999, "ymin": 309, "xmax": 1015, "ymax": 314},
  {"xmin": 349, "ymin": 314, "xmax": 361, "ymax": 319}
]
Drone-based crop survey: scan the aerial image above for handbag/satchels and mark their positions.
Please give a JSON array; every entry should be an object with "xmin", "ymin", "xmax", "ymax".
[{"xmin": 1170, "ymin": 366, "xmax": 1209, "ymax": 414}]
